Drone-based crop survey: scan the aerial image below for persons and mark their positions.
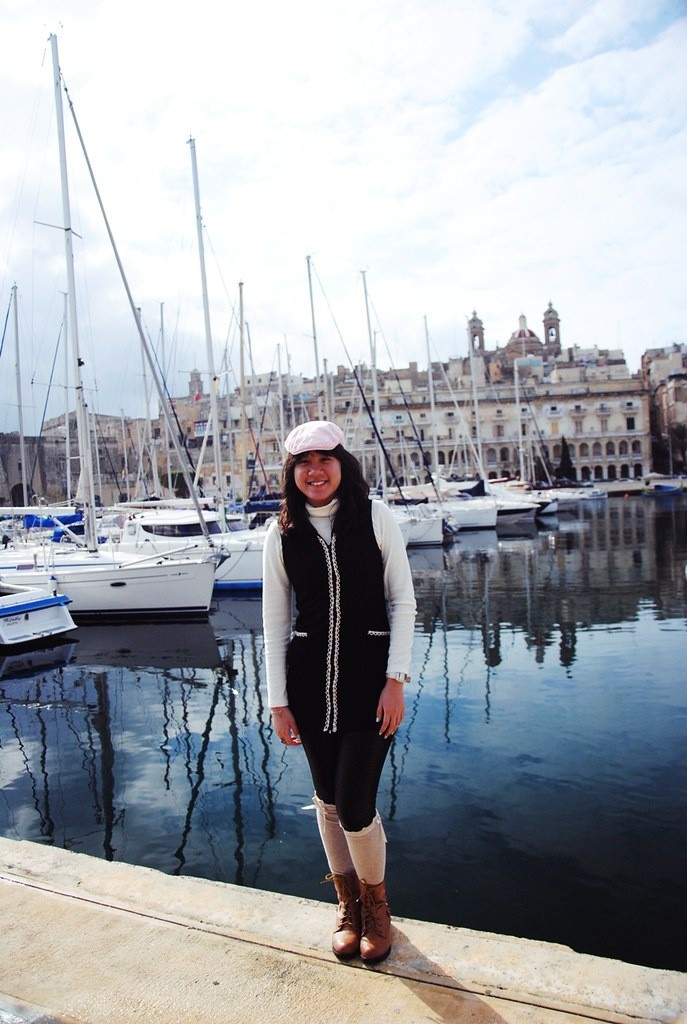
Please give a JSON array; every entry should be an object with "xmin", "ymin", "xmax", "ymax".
[{"xmin": 262, "ymin": 421, "xmax": 417, "ymax": 966}]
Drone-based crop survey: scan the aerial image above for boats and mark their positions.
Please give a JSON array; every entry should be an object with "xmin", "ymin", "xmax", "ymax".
[
  {"xmin": 1, "ymin": 581, "xmax": 79, "ymax": 648},
  {"xmin": 589, "ymin": 478, "xmax": 643, "ymax": 497},
  {"xmin": 644, "ymin": 474, "xmax": 687, "ymax": 495}
]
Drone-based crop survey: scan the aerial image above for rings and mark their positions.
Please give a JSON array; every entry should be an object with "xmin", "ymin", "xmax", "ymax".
[{"xmin": 280, "ymin": 741, "xmax": 285, "ymax": 743}]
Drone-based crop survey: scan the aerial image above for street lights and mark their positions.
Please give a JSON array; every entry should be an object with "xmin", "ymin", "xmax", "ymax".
[{"xmin": 661, "ymin": 433, "xmax": 673, "ymax": 474}]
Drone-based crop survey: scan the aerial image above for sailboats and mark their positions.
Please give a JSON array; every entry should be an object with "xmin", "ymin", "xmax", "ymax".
[
  {"xmin": 0, "ymin": 136, "xmax": 608, "ymax": 590},
  {"xmin": 0, "ymin": 33, "xmax": 231, "ymax": 614}
]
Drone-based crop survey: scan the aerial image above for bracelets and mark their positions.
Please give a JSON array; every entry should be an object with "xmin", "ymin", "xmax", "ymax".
[{"xmin": 271, "ymin": 710, "xmax": 283, "ymax": 715}]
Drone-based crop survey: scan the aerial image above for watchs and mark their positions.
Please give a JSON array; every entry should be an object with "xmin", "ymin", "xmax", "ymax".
[{"xmin": 386, "ymin": 673, "xmax": 411, "ymax": 683}]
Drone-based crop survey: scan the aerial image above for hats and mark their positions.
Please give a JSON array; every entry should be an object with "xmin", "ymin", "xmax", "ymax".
[{"xmin": 284, "ymin": 421, "xmax": 345, "ymax": 455}]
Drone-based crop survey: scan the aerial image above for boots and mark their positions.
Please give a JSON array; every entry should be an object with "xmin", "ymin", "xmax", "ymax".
[
  {"xmin": 356, "ymin": 879, "xmax": 391, "ymax": 965},
  {"xmin": 320, "ymin": 871, "xmax": 362, "ymax": 959}
]
